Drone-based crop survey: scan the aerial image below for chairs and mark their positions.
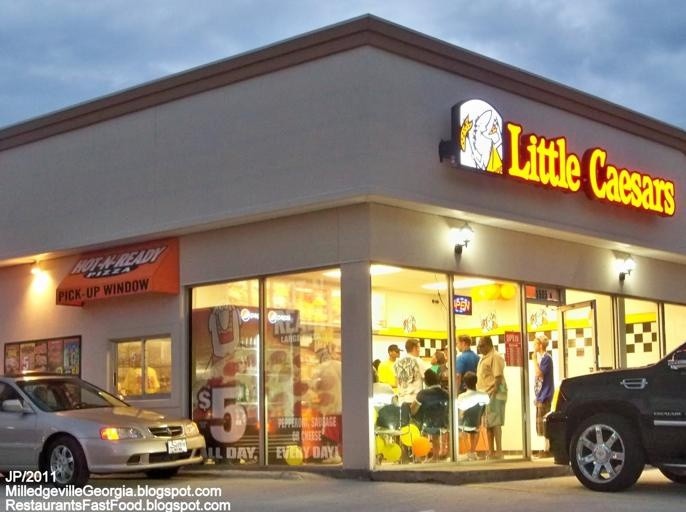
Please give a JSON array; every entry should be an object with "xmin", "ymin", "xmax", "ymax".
[
  {"xmin": 46, "ymin": 390, "xmax": 58, "ymax": 406},
  {"xmin": 376, "ymin": 402, "xmax": 487, "ymax": 465}
]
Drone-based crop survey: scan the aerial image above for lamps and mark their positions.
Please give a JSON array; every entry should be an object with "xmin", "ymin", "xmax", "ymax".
[
  {"xmin": 454, "ymin": 221, "xmax": 474, "ymax": 254},
  {"xmin": 619, "ymin": 254, "xmax": 636, "ymax": 281}
]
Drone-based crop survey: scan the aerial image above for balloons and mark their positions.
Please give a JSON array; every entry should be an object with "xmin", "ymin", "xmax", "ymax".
[{"xmin": 283, "ymin": 446, "xmax": 304, "ymax": 467}]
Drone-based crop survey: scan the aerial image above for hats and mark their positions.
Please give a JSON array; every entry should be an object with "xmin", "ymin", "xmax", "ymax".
[
  {"xmin": 388, "ymin": 345, "xmax": 403, "ymax": 352},
  {"xmin": 535, "ymin": 335, "xmax": 549, "ymax": 349}
]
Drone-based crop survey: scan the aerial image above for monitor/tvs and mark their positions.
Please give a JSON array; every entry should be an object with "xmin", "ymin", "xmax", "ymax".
[{"xmin": 453, "ymin": 295, "xmax": 472, "ymax": 315}]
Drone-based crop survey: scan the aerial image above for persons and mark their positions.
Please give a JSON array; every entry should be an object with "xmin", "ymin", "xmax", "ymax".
[
  {"xmin": 122, "ymin": 351, "xmax": 160, "ymax": 396},
  {"xmin": 310, "ymin": 341, "xmax": 343, "ymax": 463},
  {"xmin": 371, "ymin": 336, "xmax": 507, "ymax": 462},
  {"xmin": 532, "ymin": 334, "xmax": 555, "ymax": 459},
  {"xmin": 34, "ymin": 387, "xmax": 56, "ymax": 409}
]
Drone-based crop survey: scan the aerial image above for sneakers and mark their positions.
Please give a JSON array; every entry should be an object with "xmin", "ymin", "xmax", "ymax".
[{"xmin": 533, "ymin": 451, "xmax": 552, "ymax": 458}]
